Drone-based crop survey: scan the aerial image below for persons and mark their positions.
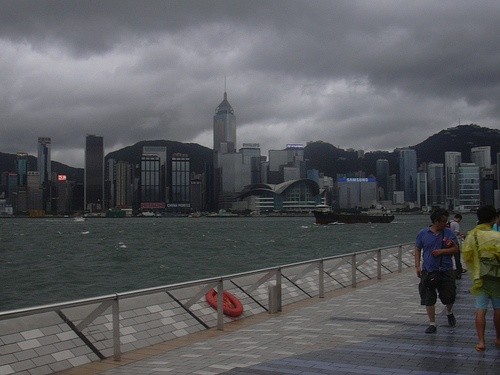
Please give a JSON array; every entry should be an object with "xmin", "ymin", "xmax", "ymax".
[
  {"xmin": 461, "ymin": 205, "xmax": 500, "ymax": 352},
  {"xmin": 415, "ymin": 208, "xmax": 459, "ymax": 334},
  {"xmin": 492, "ymin": 211, "xmax": 500, "ymax": 233},
  {"xmin": 445, "ymin": 214, "xmax": 467, "ymax": 273}
]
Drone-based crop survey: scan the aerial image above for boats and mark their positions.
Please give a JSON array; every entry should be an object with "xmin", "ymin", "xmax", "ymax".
[{"xmin": 312, "ymin": 206, "xmax": 395, "ymax": 223}]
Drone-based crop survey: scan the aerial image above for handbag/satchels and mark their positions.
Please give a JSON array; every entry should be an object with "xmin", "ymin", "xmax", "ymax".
[
  {"xmin": 424, "ymin": 270, "xmax": 441, "ymax": 288},
  {"xmin": 478, "ymin": 256, "xmax": 500, "ymax": 280}
]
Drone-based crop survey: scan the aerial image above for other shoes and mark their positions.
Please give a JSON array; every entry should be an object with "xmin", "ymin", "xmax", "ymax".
[
  {"xmin": 446, "ymin": 312, "xmax": 456, "ymax": 326},
  {"xmin": 425, "ymin": 324, "xmax": 437, "ymax": 334},
  {"xmin": 460, "ymin": 269, "xmax": 467, "ymax": 273}
]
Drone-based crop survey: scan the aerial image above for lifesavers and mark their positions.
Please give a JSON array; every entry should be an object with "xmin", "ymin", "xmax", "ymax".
[{"xmin": 207, "ymin": 289, "xmax": 244, "ymax": 317}]
